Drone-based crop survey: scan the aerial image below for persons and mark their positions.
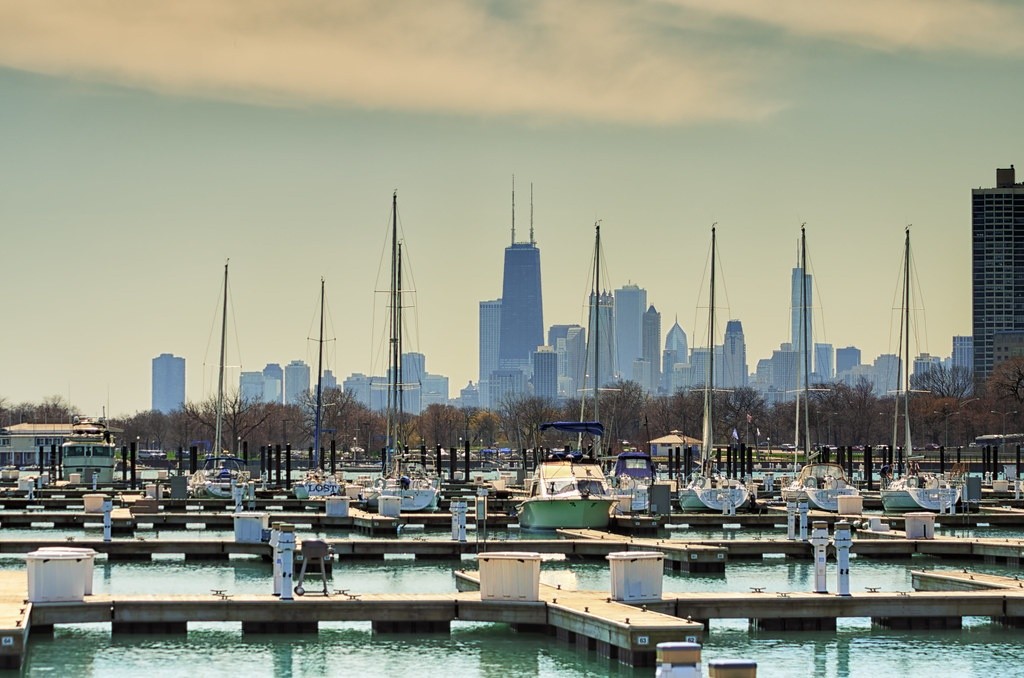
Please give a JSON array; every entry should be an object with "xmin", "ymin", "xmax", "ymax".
[{"xmin": 880, "ymin": 464, "xmax": 894, "ymax": 479}]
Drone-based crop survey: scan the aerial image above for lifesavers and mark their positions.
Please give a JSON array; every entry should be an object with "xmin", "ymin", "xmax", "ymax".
[
  {"xmin": 605, "ymin": 475, "xmax": 617, "ymax": 488},
  {"xmin": 907, "ymin": 475, "xmax": 919, "ymax": 488},
  {"xmin": 374, "ymin": 477, "xmax": 387, "ymax": 490},
  {"xmin": 804, "ymin": 476, "xmax": 817, "ymax": 488},
  {"xmin": 692, "ymin": 476, "xmax": 707, "ymax": 489}
]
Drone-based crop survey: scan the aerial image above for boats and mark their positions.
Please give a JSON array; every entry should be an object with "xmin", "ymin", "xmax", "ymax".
[
  {"xmin": 512, "ymin": 421, "xmax": 621, "ymax": 534},
  {"xmin": 61, "ymin": 405, "xmax": 115, "ymax": 485},
  {"xmin": 605, "ymin": 452, "xmax": 663, "ymax": 532}
]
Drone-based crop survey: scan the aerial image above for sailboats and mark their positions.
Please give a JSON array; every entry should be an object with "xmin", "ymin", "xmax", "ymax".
[
  {"xmin": 361, "ymin": 190, "xmax": 440, "ymax": 514},
  {"xmin": 677, "ymin": 222, "xmax": 756, "ymax": 527},
  {"xmin": 880, "ymin": 223, "xmax": 965, "ymax": 514},
  {"xmin": 185, "ymin": 260, "xmax": 250, "ymax": 503},
  {"xmin": 780, "ymin": 224, "xmax": 859, "ymax": 511},
  {"xmin": 290, "ymin": 274, "xmax": 345, "ymax": 498}
]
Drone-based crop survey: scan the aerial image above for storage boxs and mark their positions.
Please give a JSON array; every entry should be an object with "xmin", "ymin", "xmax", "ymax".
[
  {"xmin": 345, "ymin": 485, "xmax": 364, "ymax": 499},
  {"xmin": 69, "ymin": 473, "xmax": 81, "ymax": 483},
  {"xmin": 158, "ymin": 472, "xmax": 167, "ymax": 479},
  {"xmin": 325, "ymin": 495, "xmax": 351, "ymax": 517},
  {"xmin": 136, "ymin": 472, "xmax": 141, "ymax": 479},
  {"xmin": 473, "ymin": 552, "xmax": 542, "ymax": 602},
  {"xmin": 145, "ymin": 484, "xmax": 164, "ymax": 499},
  {"xmin": 836, "ymin": 494, "xmax": 865, "ymax": 515},
  {"xmin": 454, "ymin": 470, "xmax": 534, "ymax": 490},
  {"xmin": 992, "ymin": 480, "xmax": 1009, "ymax": 492},
  {"xmin": 82, "ymin": 494, "xmax": 108, "ymax": 513},
  {"xmin": 38, "ymin": 546, "xmax": 100, "ymax": 596},
  {"xmin": 22, "ymin": 551, "xmax": 92, "ymax": 603},
  {"xmin": 605, "ymin": 551, "xmax": 665, "ymax": 601},
  {"xmin": 230, "ymin": 511, "xmax": 269, "ymax": 543},
  {"xmin": 2, "ymin": 470, "xmax": 51, "ymax": 490},
  {"xmin": 902, "ymin": 512, "xmax": 937, "ymax": 539},
  {"xmin": 377, "ymin": 495, "xmax": 403, "ymax": 516}
]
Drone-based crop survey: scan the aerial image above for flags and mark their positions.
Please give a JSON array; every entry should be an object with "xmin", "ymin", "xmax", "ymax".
[
  {"xmin": 733, "ymin": 429, "xmax": 738, "ymax": 439},
  {"xmin": 757, "ymin": 429, "xmax": 760, "ymax": 436},
  {"xmin": 747, "ymin": 415, "xmax": 751, "ymax": 423}
]
